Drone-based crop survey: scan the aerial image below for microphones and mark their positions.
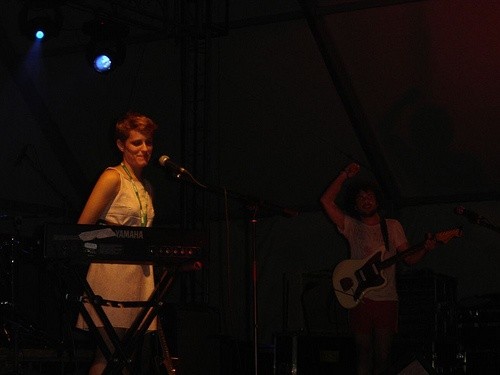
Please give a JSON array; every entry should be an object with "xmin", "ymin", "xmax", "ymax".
[
  {"xmin": 159, "ymin": 155, "xmax": 187, "ymax": 174},
  {"xmin": 456, "ymin": 206, "xmax": 488, "ymax": 220}
]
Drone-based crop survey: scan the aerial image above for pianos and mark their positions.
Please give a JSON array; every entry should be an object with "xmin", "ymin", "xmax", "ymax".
[{"xmin": 48, "ymin": 220, "xmax": 202, "ymax": 375}]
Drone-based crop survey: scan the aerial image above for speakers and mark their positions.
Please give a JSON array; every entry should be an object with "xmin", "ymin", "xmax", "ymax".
[{"xmin": 391, "ymin": 355, "xmax": 428, "ymax": 375}]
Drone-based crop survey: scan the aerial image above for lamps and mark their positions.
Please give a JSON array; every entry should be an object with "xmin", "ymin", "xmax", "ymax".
[
  {"xmin": 81, "ymin": 20, "xmax": 130, "ymax": 75},
  {"xmin": 17, "ymin": 2, "xmax": 63, "ymax": 42}
]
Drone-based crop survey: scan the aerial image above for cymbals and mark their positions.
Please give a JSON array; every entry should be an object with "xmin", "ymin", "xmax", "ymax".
[{"xmin": 0, "ymin": 197, "xmax": 57, "ymax": 216}]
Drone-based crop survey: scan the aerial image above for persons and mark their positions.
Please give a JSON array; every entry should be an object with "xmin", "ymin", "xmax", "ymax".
[
  {"xmin": 77, "ymin": 114, "xmax": 157, "ymax": 375},
  {"xmin": 322, "ymin": 163, "xmax": 438, "ymax": 375}
]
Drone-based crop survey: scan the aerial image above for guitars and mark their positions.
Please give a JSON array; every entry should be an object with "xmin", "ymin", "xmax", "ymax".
[{"xmin": 332, "ymin": 229, "xmax": 463, "ymax": 309}]
